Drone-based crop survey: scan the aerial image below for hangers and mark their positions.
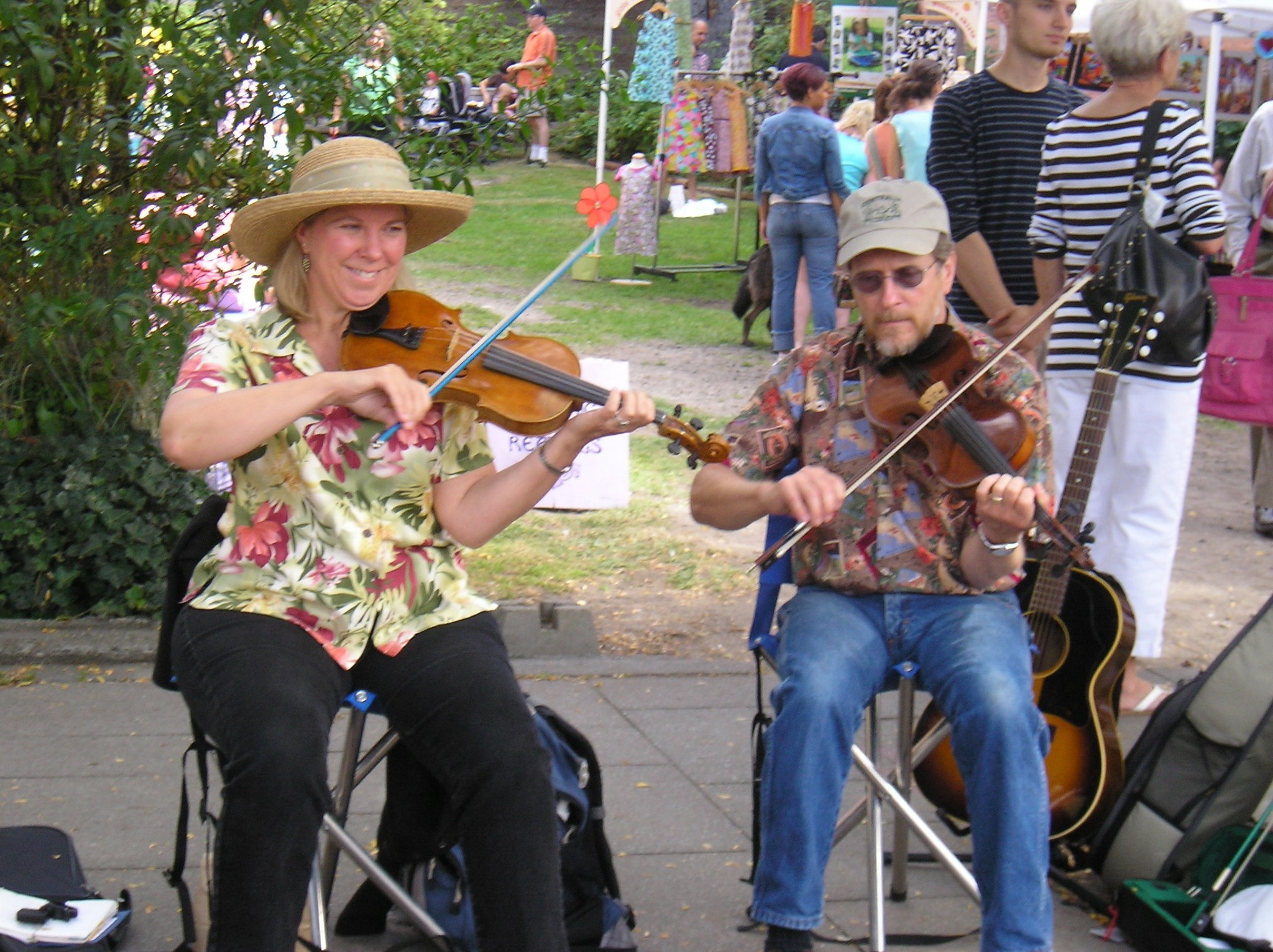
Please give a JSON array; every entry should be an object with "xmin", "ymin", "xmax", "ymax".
[
  {"xmin": 636, "ymin": 0, "xmax": 681, "ymax": 22},
  {"xmin": 672, "ymin": 71, "xmax": 844, "ymax": 100},
  {"xmin": 730, "ymin": 0, "xmax": 752, "ymax": 12}
]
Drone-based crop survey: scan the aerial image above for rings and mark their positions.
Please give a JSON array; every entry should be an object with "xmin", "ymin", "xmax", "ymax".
[
  {"xmin": 616, "ymin": 411, "xmax": 630, "ymax": 428},
  {"xmin": 988, "ymin": 494, "xmax": 1002, "ymax": 502}
]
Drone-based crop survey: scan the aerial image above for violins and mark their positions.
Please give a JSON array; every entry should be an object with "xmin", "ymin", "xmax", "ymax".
[
  {"xmin": 860, "ymin": 322, "xmax": 1096, "ymax": 580},
  {"xmin": 339, "ymin": 289, "xmax": 732, "ymax": 471}
]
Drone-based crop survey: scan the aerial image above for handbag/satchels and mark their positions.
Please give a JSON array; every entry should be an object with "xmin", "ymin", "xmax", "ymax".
[
  {"xmin": 1194, "ymin": 185, "xmax": 1273, "ymax": 425},
  {"xmin": 1082, "ymin": 101, "xmax": 1217, "ymax": 366}
]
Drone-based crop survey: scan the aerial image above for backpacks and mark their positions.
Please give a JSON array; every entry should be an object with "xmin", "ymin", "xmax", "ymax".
[
  {"xmin": 383, "ymin": 692, "xmax": 640, "ymax": 951},
  {"xmin": 1085, "ymin": 594, "xmax": 1273, "ymax": 906}
]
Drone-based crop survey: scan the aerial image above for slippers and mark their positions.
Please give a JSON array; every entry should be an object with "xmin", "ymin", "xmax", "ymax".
[{"xmin": 1117, "ymin": 684, "xmax": 1174, "ymax": 716}]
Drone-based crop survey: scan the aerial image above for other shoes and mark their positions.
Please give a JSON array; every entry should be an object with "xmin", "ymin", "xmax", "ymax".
[
  {"xmin": 1255, "ymin": 505, "xmax": 1273, "ymax": 533},
  {"xmin": 522, "ymin": 159, "xmax": 550, "ymax": 168}
]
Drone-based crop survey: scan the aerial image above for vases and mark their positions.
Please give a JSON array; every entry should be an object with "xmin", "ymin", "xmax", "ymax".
[{"xmin": 571, "ymin": 251, "xmax": 603, "ymax": 281}]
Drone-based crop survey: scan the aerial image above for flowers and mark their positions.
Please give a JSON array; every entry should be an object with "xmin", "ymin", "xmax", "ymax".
[{"xmin": 576, "ymin": 183, "xmax": 618, "ymax": 228}]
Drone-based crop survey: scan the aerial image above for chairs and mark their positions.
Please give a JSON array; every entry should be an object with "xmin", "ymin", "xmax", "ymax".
[
  {"xmin": 745, "ymin": 455, "xmax": 982, "ymax": 952},
  {"xmin": 152, "ymin": 496, "xmax": 455, "ymax": 952}
]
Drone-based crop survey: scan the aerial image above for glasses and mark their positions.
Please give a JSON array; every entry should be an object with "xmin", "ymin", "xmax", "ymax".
[{"xmin": 848, "ymin": 258, "xmax": 940, "ymax": 293}]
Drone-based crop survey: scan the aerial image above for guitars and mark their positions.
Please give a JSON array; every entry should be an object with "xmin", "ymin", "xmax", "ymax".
[{"xmin": 912, "ymin": 289, "xmax": 1165, "ymax": 844}]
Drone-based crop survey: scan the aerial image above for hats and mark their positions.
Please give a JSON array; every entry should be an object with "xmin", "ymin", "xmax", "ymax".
[
  {"xmin": 523, "ymin": 5, "xmax": 548, "ymax": 16},
  {"xmin": 836, "ymin": 176, "xmax": 952, "ymax": 267},
  {"xmin": 231, "ymin": 136, "xmax": 472, "ymax": 267},
  {"xmin": 426, "ymin": 71, "xmax": 438, "ymax": 82}
]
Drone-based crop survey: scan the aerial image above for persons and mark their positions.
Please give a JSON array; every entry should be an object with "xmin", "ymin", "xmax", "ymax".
[
  {"xmin": 220, "ymin": 5, "xmax": 558, "ymax": 166},
  {"xmin": 689, "ymin": 178, "xmax": 1054, "ymax": 952},
  {"xmin": 155, "ymin": 134, "xmax": 656, "ymax": 949},
  {"xmin": 847, "ymin": 18, "xmax": 881, "ymax": 68},
  {"xmin": 1027, "ymin": 0, "xmax": 1230, "ymax": 721},
  {"xmin": 778, "ymin": 24, "xmax": 830, "ymax": 122},
  {"xmin": 682, "ymin": 16, "xmax": 711, "ymax": 204},
  {"xmin": 922, "ymin": 1, "xmax": 1092, "ymax": 372},
  {"xmin": 1216, "ymin": 99, "xmax": 1273, "ymax": 543},
  {"xmin": 753, "ymin": 58, "xmax": 951, "ymax": 361}
]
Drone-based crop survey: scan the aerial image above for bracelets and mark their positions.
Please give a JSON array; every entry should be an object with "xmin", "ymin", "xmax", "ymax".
[{"xmin": 540, "ymin": 443, "xmax": 572, "ymax": 475}]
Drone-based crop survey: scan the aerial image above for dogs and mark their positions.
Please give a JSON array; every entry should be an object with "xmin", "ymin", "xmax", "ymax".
[{"xmin": 727, "ymin": 241, "xmax": 776, "ymax": 348}]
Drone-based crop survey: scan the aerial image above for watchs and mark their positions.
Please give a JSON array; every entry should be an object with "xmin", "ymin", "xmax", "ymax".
[{"xmin": 977, "ymin": 524, "xmax": 1021, "ymax": 558}]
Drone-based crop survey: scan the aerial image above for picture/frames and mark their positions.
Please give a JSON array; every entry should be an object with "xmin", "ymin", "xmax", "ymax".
[
  {"xmin": 1216, "ymin": 50, "xmax": 1262, "ymax": 121},
  {"xmin": 1072, "ymin": 42, "xmax": 1113, "ymax": 91},
  {"xmin": 1159, "ymin": 48, "xmax": 1209, "ymax": 102},
  {"xmin": 1047, "ymin": 36, "xmax": 1077, "ymax": 84}
]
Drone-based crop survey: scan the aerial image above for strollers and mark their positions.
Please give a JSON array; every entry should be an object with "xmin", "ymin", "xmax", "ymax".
[{"xmin": 418, "ymin": 70, "xmax": 490, "ymax": 167}]
